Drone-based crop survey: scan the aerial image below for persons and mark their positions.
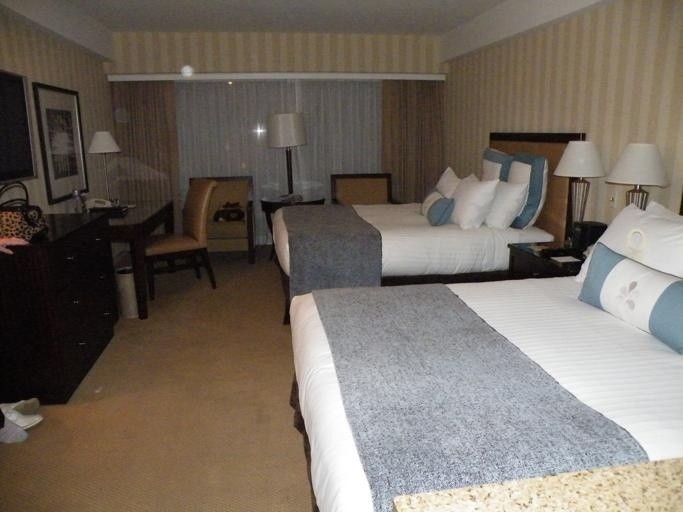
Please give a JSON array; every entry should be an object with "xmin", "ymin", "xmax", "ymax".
[{"xmin": 0, "ymin": 237, "xmax": 29, "ymax": 444}]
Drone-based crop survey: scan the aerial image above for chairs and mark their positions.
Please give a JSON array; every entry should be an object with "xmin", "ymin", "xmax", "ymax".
[
  {"xmin": 330, "ymin": 173, "xmax": 401, "ymax": 205},
  {"xmin": 143, "ymin": 178, "xmax": 218, "ymax": 299},
  {"xmin": 183, "ymin": 176, "xmax": 254, "ymax": 265}
]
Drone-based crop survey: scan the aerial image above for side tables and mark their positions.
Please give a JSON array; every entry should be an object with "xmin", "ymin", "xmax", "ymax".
[{"xmin": 261, "ymin": 194, "xmax": 325, "ymax": 261}]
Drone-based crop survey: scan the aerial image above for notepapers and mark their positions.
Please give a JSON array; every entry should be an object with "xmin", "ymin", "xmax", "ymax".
[{"xmin": 550, "ymin": 255, "xmax": 581, "ymax": 263}]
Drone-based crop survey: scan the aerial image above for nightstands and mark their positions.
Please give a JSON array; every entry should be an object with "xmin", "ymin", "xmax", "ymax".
[{"xmin": 508, "ymin": 242, "xmax": 586, "ymax": 281}]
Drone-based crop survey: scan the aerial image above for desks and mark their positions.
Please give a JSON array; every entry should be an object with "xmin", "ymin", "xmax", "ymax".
[{"xmin": 83, "ymin": 201, "xmax": 174, "ymax": 320}]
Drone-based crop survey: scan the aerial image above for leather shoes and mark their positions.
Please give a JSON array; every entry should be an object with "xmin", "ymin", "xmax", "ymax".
[
  {"xmin": 4, "ymin": 410, "xmax": 43, "ymax": 429},
  {"xmin": 0, "ymin": 398, "xmax": 40, "ymax": 415}
]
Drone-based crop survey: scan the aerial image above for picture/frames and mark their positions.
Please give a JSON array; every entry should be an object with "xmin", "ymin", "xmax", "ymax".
[{"xmin": 32, "ymin": 81, "xmax": 90, "ymax": 206}]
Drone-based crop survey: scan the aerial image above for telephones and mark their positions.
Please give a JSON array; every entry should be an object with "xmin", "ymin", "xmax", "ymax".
[
  {"xmin": 84, "ymin": 197, "xmax": 113, "ymax": 210},
  {"xmin": 279, "ymin": 192, "xmax": 304, "ymax": 203}
]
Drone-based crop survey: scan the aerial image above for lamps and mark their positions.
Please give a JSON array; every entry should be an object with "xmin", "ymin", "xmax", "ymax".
[
  {"xmin": 88, "ymin": 132, "xmax": 122, "ymax": 208},
  {"xmin": 553, "ymin": 141, "xmax": 606, "ymax": 221},
  {"xmin": 268, "ymin": 113, "xmax": 308, "ymax": 199},
  {"xmin": 605, "ymin": 143, "xmax": 670, "ymax": 211}
]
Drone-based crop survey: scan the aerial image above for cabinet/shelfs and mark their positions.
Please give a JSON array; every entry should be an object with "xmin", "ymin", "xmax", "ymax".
[{"xmin": 0, "ymin": 212, "xmax": 121, "ymax": 405}]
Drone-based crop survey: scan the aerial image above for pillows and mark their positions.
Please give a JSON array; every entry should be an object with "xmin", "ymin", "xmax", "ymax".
[
  {"xmin": 578, "ymin": 243, "xmax": 683, "ymax": 356},
  {"xmin": 499, "ymin": 153, "xmax": 549, "ymax": 230},
  {"xmin": 420, "ymin": 189, "xmax": 454, "ymax": 226},
  {"xmin": 462, "ymin": 173, "xmax": 480, "ymax": 182},
  {"xmin": 436, "ymin": 166, "xmax": 460, "ymax": 198},
  {"xmin": 485, "ymin": 181, "xmax": 528, "ymax": 230},
  {"xmin": 575, "ymin": 203, "xmax": 683, "ymax": 284},
  {"xmin": 646, "ymin": 202, "xmax": 683, "ymax": 224},
  {"xmin": 447, "ymin": 181, "xmax": 499, "ymax": 230}
]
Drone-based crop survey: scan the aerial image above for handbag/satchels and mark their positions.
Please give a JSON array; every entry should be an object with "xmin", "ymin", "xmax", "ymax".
[{"xmin": 0, "ymin": 180, "xmax": 49, "ymax": 242}]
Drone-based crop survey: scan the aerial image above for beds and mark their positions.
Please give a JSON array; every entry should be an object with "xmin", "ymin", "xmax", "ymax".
[
  {"xmin": 273, "ymin": 132, "xmax": 586, "ymax": 326},
  {"xmin": 290, "ymin": 189, "xmax": 683, "ymax": 512}
]
